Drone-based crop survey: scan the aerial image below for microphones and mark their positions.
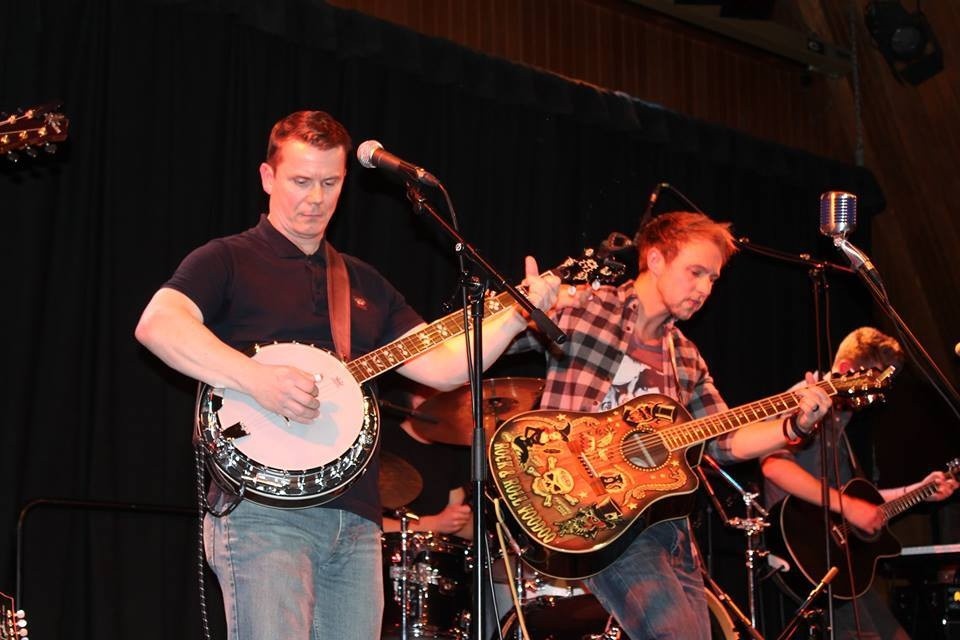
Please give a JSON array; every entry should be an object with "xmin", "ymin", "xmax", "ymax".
[
  {"xmin": 632, "ymin": 188, "xmax": 659, "ymax": 247},
  {"xmin": 818, "ymin": 189, "xmax": 882, "ymax": 282},
  {"xmin": 356, "ymin": 138, "xmax": 442, "ymax": 189}
]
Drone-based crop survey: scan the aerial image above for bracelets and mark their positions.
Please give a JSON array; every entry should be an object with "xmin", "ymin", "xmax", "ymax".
[
  {"xmin": 783, "ymin": 416, "xmax": 804, "ymax": 446},
  {"xmin": 790, "ymin": 413, "xmax": 819, "ymax": 439},
  {"xmin": 902, "ymin": 485, "xmax": 907, "ymax": 494}
]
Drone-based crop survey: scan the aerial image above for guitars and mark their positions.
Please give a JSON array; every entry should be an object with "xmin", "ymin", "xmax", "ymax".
[
  {"xmin": 193, "ymin": 229, "xmax": 634, "ymax": 510},
  {"xmin": 0, "ymin": 589, "xmax": 29, "ymax": 640},
  {"xmin": 1, "ymin": 107, "xmax": 68, "ymax": 165},
  {"xmin": 487, "ymin": 361, "xmax": 898, "ymax": 581},
  {"xmin": 762, "ymin": 456, "xmax": 960, "ymax": 612}
]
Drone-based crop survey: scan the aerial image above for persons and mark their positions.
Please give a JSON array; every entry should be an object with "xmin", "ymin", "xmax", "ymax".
[
  {"xmin": 134, "ymin": 112, "xmax": 562, "ymax": 640},
  {"xmin": 759, "ymin": 327, "xmax": 960, "ymax": 640},
  {"xmin": 379, "ymin": 381, "xmax": 472, "ymax": 539},
  {"xmin": 503, "ymin": 212, "xmax": 833, "ymax": 640}
]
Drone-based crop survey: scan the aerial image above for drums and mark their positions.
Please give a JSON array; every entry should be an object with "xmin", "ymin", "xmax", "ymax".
[{"xmin": 381, "ymin": 529, "xmax": 478, "ymax": 640}]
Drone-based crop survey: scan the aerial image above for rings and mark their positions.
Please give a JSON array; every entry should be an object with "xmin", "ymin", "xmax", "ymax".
[{"xmin": 812, "ymin": 404, "xmax": 819, "ymax": 411}]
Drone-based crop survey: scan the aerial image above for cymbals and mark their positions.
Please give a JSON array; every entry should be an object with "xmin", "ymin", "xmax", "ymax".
[
  {"xmin": 410, "ymin": 377, "xmax": 545, "ymax": 447},
  {"xmin": 378, "ymin": 451, "xmax": 424, "ymax": 510}
]
